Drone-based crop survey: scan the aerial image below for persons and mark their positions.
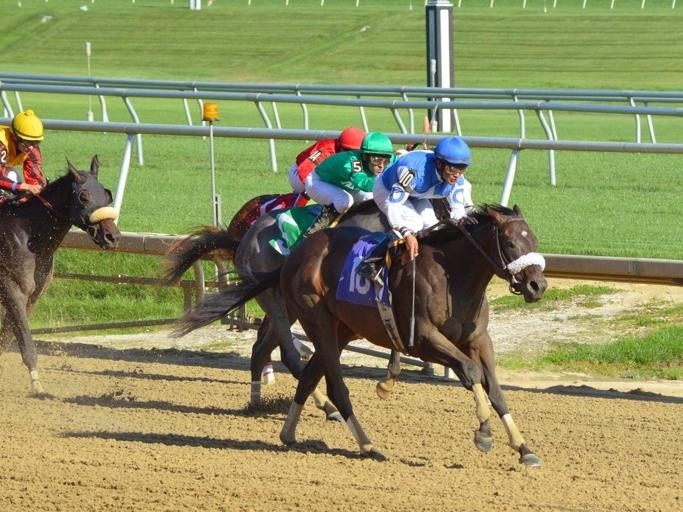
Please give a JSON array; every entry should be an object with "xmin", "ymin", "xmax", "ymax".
[
  {"xmin": 289, "ymin": 127, "xmax": 366, "ymax": 199},
  {"xmin": 0, "ymin": 109, "xmax": 48, "ymax": 205},
  {"xmin": 302, "ymin": 131, "xmax": 403, "ymax": 239},
  {"xmin": 356, "ymin": 137, "xmax": 474, "ymax": 287}
]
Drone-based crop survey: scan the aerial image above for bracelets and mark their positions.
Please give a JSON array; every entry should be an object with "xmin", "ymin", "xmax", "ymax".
[{"xmin": 12, "ymin": 181, "xmax": 17, "ymax": 193}]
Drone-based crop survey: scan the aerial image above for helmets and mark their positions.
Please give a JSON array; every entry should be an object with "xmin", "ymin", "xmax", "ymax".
[
  {"xmin": 435, "ymin": 137, "xmax": 472, "ymax": 166},
  {"xmin": 10, "ymin": 109, "xmax": 43, "ymax": 141},
  {"xmin": 361, "ymin": 131, "xmax": 393, "ymax": 155},
  {"xmin": 340, "ymin": 128, "xmax": 365, "ymax": 150}
]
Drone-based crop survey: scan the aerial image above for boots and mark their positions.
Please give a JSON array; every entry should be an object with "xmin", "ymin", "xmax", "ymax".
[
  {"xmin": 303, "ymin": 204, "xmax": 340, "ymax": 238},
  {"xmin": 354, "ymin": 238, "xmax": 391, "ymax": 288}
]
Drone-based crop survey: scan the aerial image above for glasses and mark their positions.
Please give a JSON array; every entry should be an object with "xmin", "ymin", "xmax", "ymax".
[
  {"xmin": 445, "ymin": 163, "xmax": 466, "ymax": 174},
  {"xmin": 20, "ymin": 140, "xmax": 40, "ymax": 147},
  {"xmin": 369, "ymin": 155, "xmax": 389, "ymax": 165}
]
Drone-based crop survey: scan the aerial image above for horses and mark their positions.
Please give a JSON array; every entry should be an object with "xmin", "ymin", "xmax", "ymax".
[
  {"xmin": 167, "ymin": 142, "xmax": 434, "ymax": 384},
  {"xmin": 166, "ymin": 202, "xmax": 549, "ymax": 469},
  {"xmin": 163, "ymin": 199, "xmax": 401, "ymax": 422},
  {"xmin": 0, "ymin": 153, "xmax": 122, "ymax": 401}
]
